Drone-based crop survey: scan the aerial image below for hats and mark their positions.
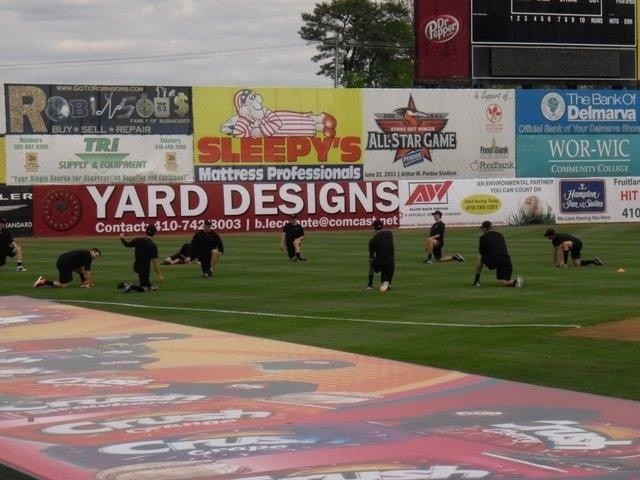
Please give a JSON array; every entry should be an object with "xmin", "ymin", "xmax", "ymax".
[
  {"xmin": 372, "ymin": 220, "xmax": 384, "ymax": 230},
  {"xmin": 544, "ymin": 228, "xmax": 555, "ymax": 236},
  {"xmin": 431, "ymin": 211, "xmax": 443, "ymax": 215},
  {"xmin": 146, "ymin": 224, "xmax": 157, "ymax": 232},
  {"xmin": 480, "ymin": 221, "xmax": 492, "ymax": 228},
  {"xmin": 204, "ymin": 220, "xmax": 213, "ymax": 225},
  {"xmin": 289, "ymin": 213, "xmax": 299, "ymax": 218},
  {"xmin": 0, "ymin": 217, "xmax": 9, "ymax": 223}
]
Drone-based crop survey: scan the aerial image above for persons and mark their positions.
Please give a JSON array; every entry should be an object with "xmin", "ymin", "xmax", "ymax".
[
  {"xmin": 364, "ymin": 218, "xmax": 396, "ymax": 292},
  {"xmin": 543, "ymin": 228, "xmax": 603, "ymax": 271},
  {"xmin": 423, "ymin": 210, "xmax": 464, "ymax": 264},
  {"xmin": 32, "ymin": 247, "xmax": 103, "ymax": 290},
  {"xmin": 472, "ymin": 220, "xmax": 524, "ymax": 290},
  {"xmin": 191, "ymin": 221, "xmax": 225, "ymax": 279},
  {"xmin": 280, "ymin": 212, "xmax": 305, "ymax": 262},
  {"xmin": 0, "ymin": 218, "xmax": 27, "ymax": 272},
  {"xmin": 117, "ymin": 224, "xmax": 164, "ymax": 295},
  {"xmin": 160, "ymin": 243, "xmax": 196, "ymax": 266}
]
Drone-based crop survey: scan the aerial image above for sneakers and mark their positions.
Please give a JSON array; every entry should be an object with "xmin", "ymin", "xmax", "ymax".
[
  {"xmin": 80, "ymin": 280, "xmax": 96, "ymax": 288},
  {"xmin": 560, "ymin": 264, "xmax": 569, "ymax": 271},
  {"xmin": 456, "ymin": 253, "xmax": 464, "ymax": 262},
  {"xmin": 201, "ymin": 271, "xmax": 216, "ymax": 279},
  {"xmin": 33, "ymin": 275, "xmax": 47, "ymax": 289},
  {"xmin": 161, "ymin": 260, "xmax": 171, "ymax": 265},
  {"xmin": 513, "ymin": 275, "xmax": 522, "ymax": 288},
  {"xmin": 16, "ymin": 265, "xmax": 27, "ymax": 272},
  {"xmin": 472, "ymin": 281, "xmax": 481, "ymax": 288},
  {"xmin": 116, "ymin": 281, "xmax": 161, "ymax": 294},
  {"xmin": 594, "ymin": 256, "xmax": 603, "ymax": 265},
  {"xmin": 319, "ymin": 111, "xmax": 337, "ymax": 139},
  {"xmin": 421, "ymin": 259, "xmax": 433, "ymax": 264},
  {"xmin": 363, "ymin": 281, "xmax": 389, "ymax": 292},
  {"xmin": 296, "ymin": 256, "xmax": 307, "ymax": 264}
]
